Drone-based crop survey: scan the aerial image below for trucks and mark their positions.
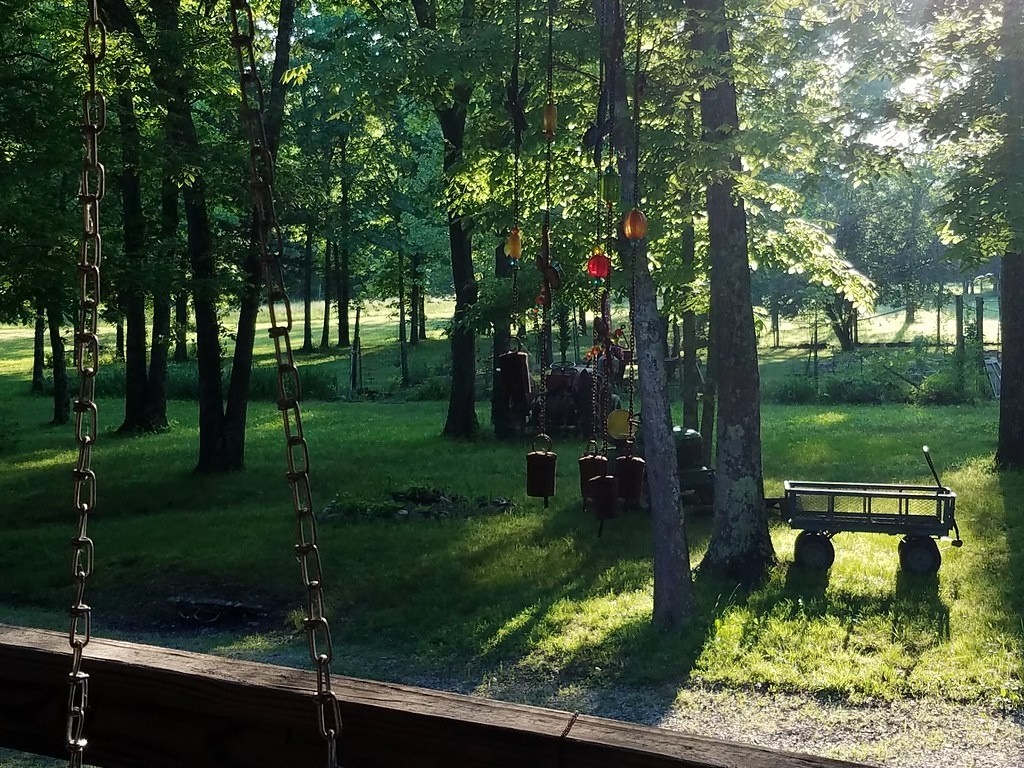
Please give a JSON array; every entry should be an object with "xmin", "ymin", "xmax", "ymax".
[{"xmin": 627, "ymin": 425, "xmax": 962, "ymax": 574}]
